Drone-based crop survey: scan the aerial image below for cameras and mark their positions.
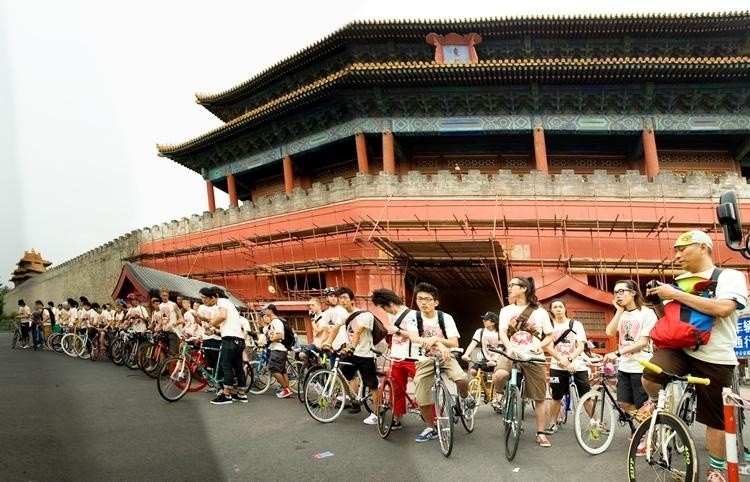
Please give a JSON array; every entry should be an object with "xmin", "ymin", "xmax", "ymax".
[{"xmin": 646, "ymin": 280, "xmax": 660, "ymax": 305}]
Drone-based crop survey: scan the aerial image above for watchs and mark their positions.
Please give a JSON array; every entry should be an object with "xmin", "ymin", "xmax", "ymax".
[
  {"xmin": 614, "ymin": 350, "xmax": 621, "ymax": 357},
  {"xmin": 396, "ymin": 328, "xmax": 400, "ymax": 335},
  {"xmin": 568, "ymin": 357, "xmax": 572, "ymax": 361}
]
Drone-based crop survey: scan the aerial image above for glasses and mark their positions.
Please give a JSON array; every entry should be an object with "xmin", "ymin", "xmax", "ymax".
[
  {"xmin": 416, "ymin": 296, "xmax": 438, "ymax": 302},
  {"xmin": 612, "ymin": 288, "xmax": 631, "ymax": 296},
  {"xmin": 508, "ymin": 283, "xmax": 522, "ymax": 287},
  {"xmin": 380, "ymin": 304, "xmax": 385, "ymax": 308}
]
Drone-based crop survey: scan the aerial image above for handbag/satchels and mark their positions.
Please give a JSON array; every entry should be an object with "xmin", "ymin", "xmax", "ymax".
[
  {"xmin": 345, "ymin": 310, "xmax": 387, "ymax": 346},
  {"xmin": 649, "ymin": 267, "xmax": 723, "ymax": 347},
  {"xmin": 387, "ymin": 309, "xmax": 412, "ymax": 349}
]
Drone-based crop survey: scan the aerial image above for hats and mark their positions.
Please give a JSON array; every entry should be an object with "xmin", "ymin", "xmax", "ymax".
[
  {"xmin": 481, "ymin": 312, "xmax": 499, "ymax": 323},
  {"xmin": 673, "ymin": 230, "xmax": 713, "ymax": 250}
]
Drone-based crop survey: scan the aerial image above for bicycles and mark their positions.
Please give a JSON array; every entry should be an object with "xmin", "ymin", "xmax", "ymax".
[
  {"xmin": 412, "ymin": 343, "xmax": 488, "ymax": 457},
  {"xmin": 552, "ymin": 361, "xmax": 582, "ymax": 444},
  {"xmin": 463, "ymin": 360, "xmax": 511, "ymax": 421},
  {"xmin": 574, "ymin": 338, "xmax": 646, "ymax": 456},
  {"xmin": 369, "ymin": 347, "xmax": 448, "ymax": 445},
  {"xmin": 243, "ymin": 321, "xmax": 336, "ymax": 407},
  {"xmin": 487, "ymin": 347, "xmax": 547, "ymax": 463},
  {"xmin": 157, "ymin": 327, "xmax": 255, "ymax": 403},
  {"xmin": 626, "ymin": 358, "xmax": 711, "ymax": 482},
  {"xmin": 11, "ymin": 314, "xmax": 210, "ymax": 391},
  {"xmin": 303, "ymin": 343, "xmax": 393, "ymax": 424}
]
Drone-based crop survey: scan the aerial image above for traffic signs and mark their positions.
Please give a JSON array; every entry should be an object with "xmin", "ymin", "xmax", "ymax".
[{"xmin": 733, "ymin": 312, "xmax": 750, "ymax": 359}]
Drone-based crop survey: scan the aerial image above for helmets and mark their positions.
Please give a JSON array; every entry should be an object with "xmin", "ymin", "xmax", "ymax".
[
  {"xmin": 258, "ymin": 310, "xmax": 268, "ymax": 317},
  {"xmin": 115, "ymin": 299, "xmax": 126, "ymax": 305},
  {"xmin": 127, "ymin": 294, "xmax": 137, "ymax": 300},
  {"xmin": 324, "ymin": 287, "xmax": 336, "ymax": 296}
]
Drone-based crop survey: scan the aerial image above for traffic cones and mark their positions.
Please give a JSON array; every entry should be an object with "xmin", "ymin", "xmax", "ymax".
[{"xmin": 722, "ymin": 387, "xmax": 740, "ymax": 482}]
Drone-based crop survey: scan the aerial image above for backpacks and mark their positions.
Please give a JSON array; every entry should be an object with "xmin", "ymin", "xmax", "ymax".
[
  {"xmin": 273, "ymin": 317, "xmax": 295, "ymax": 352},
  {"xmin": 41, "ymin": 308, "xmax": 55, "ymax": 328}
]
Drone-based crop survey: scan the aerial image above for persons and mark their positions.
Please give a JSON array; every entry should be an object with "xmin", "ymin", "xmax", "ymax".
[
  {"xmin": 321, "ymin": 287, "xmax": 382, "ymax": 425},
  {"xmin": 54, "ymin": 296, "xmax": 96, "ymax": 355},
  {"xmin": 193, "ymin": 300, "xmax": 202, "ymax": 311},
  {"xmin": 16, "ymin": 299, "xmax": 31, "ymax": 349},
  {"xmin": 36, "ymin": 300, "xmax": 54, "ymax": 350},
  {"xmin": 124, "ymin": 293, "xmax": 151, "ymax": 368},
  {"xmin": 492, "ymin": 277, "xmax": 553, "ymax": 447},
  {"xmin": 322, "ymin": 288, "xmax": 351, "ymax": 410},
  {"xmin": 462, "ymin": 312, "xmax": 505, "ymax": 389},
  {"xmin": 204, "ymin": 287, "xmax": 248, "ymax": 406},
  {"xmin": 544, "ymin": 298, "xmax": 611, "ymax": 435},
  {"xmin": 182, "ymin": 298, "xmax": 202, "ymax": 350},
  {"xmin": 103, "ymin": 304, "xmax": 117, "ymax": 326},
  {"xmin": 192, "ymin": 287, "xmax": 223, "ymax": 392},
  {"xmin": 155, "ymin": 288, "xmax": 185, "ymax": 375},
  {"xmin": 634, "ymin": 229, "xmax": 748, "ymax": 482},
  {"xmin": 299, "ymin": 297, "xmax": 331, "ymax": 391},
  {"xmin": 90, "ymin": 304, "xmax": 113, "ymax": 356},
  {"xmin": 176, "ymin": 294, "xmax": 185, "ymax": 315},
  {"xmin": 372, "ymin": 287, "xmax": 443, "ymax": 430},
  {"xmin": 235, "ymin": 306, "xmax": 251, "ymax": 379},
  {"xmin": 292, "ymin": 328, "xmax": 301, "ymax": 376},
  {"xmin": 406, "ymin": 283, "xmax": 478, "ymax": 443},
  {"xmin": 265, "ymin": 304, "xmax": 295, "ymax": 397},
  {"xmin": 258, "ymin": 311, "xmax": 272, "ymax": 372},
  {"xmin": 115, "ymin": 305, "xmax": 124, "ymax": 330},
  {"xmin": 582, "ymin": 341, "xmax": 602, "ymax": 387},
  {"xmin": 603, "ymin": 280, "xmax": 658, "ymax": 457}
]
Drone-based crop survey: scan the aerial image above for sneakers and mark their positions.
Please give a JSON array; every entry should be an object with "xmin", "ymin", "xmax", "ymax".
[
  {"xmin": 23, "ymin": 346, "xmax": 30, "ymax": 348},
  {"xmin": 385, "ymin": 420, "xmax": 401, "ymax": 430},
  {"xmin": 211, "ymin": 393, "xmax": 232, "ymax": 405},
  {"xmin": 706, "ymin": 468, "xmax": 725, "ymax": 482},
  {"xmin": 635, "ymin": 441, "xmax": 656, "ymax": 456},
  {"xmin": 278, "ymin": 388, "xmax": 293, "ymax": 398},
  {"xmin": 350, "ymin": 404, "xmax": 360, "ymax": 412},
  {"xmin": 462, "ymin": 393, "xmax": 475, "ymax": 409},
  {"xmin": 20, "ymin": 345, "xmax": 24, "ymax": 347},
  {"xmin": 338, "ymin": 394, "xmax": 351, "ymax": 403},
  {"xmin": 536, "ymin": 434, "xmax": 550, "ymax": 447},
  {"xmin": 363, "ymin": 413, "xmax": 382, "ymax": 424},
  {"xmin": 494, "ymin": 393, "xmax": 504, "ymax": 409},
  {"xmin": 544, "ymin": 424, "xmax": 556, "ymax": 434},
  {"xmin": 637, "ymin": 399, "xmax": 660, "ymax": 419},
  {"xmin": 276, "ymin": 391, "xmax": 284, "ymax": 396},
  {"xmin": 415, "ymin": 428, "xmax": 438, "ymax": 442},
  {"xmin": 592, "ymin": 421, "xmax": 610, "ymax": 434},
  {"xmin": 232, "ymin": 393, "xmax": 248, "ymax": 403}
]
